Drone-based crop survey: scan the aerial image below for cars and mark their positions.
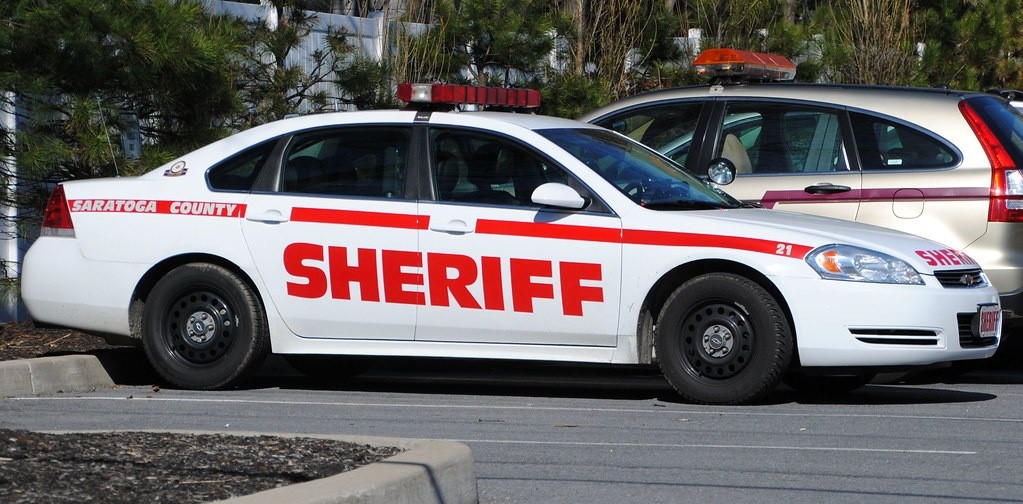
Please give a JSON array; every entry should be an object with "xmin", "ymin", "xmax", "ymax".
[{"xmin": 20, "ymin": 83, "xmax": 1004, "ymax": 406}]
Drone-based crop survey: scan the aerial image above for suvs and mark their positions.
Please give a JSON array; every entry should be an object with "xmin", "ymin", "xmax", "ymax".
[{"xmin": 435, "ymin": 49, "xmax": 1023, "ymax": 386}]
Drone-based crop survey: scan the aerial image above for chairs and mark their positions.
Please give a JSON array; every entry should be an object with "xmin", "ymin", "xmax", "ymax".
[
  {"xmin": 435, "ymin": 141, "xmax": 521, "ymax": 205},
  {"xmin": 282, "ymin": 155, "xmax": 340, "ymax": 194},
  {"xmin": 722, "ymin": 131, "xmax": 753, "ymax": 174},
  {"xmin": 838, "ymin": 124, "xmax": 883, "ymax": 171}
]
